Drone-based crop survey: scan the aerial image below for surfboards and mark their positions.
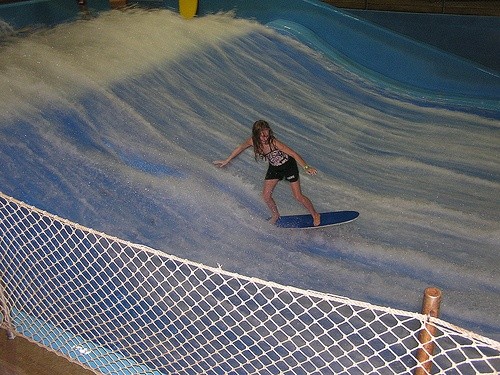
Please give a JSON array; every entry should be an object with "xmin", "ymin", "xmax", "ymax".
[
  {"xmin": 179, "ymin": 0, "xmax": 197, "ymax": 20},
  {"xmin": 264, "ymin": 210, "xmax": 360, "ymax": 229}
]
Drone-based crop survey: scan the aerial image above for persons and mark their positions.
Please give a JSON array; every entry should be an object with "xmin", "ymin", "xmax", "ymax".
[{"xmin": 213, "ymin": 120, "xmax": 321, "ymax": 227}]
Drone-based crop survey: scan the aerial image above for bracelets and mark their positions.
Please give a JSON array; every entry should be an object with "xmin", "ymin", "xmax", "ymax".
[{"xmin": 303, "ymin": 165, "xmax": 311, "ymax": 170}]
